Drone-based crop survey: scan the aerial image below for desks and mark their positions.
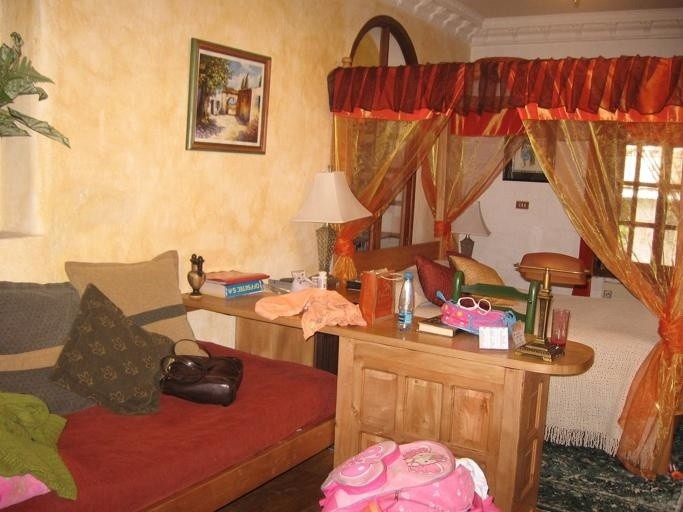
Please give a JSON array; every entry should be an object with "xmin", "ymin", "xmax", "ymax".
[{"xmin": 177, "ymin": 262, "xmax": 595, "ymax": 512}]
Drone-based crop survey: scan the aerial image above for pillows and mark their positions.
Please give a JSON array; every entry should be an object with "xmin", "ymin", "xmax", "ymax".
[
  {"xmin": 0, "ymin": 273, "xmax": 99, "ymax": 415},
  {"xmin": 65, "ymin": 247, "xmax": 194, "ymax": 341},
  {"xmin": 389, "ymin": 258, "xmax": 451, "ymax": 314},
  {"xmin": 414, "ymin": 254, "xmax": 462, "ymax": 306},
  {"xmin": 52, "ymin": 280, "xmax": 178, "ymax": 414},
  {"xmin": 445, "ymin": 252, "xmax": 501, "ymax": 287}
]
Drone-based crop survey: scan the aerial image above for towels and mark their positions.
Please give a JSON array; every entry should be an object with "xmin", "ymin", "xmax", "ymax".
[{"xmin": 258, "ymin": 285, "xmax": 367, "ymax": 339}]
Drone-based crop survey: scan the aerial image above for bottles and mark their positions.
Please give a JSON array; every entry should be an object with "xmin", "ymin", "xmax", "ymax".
[
  {"xmin": 186, "ymin": 254, "xmax": 204, "ymax": 296},
  {"xmin": 398, "ymin": 272, "xmax": 412, "ymax": 333}
]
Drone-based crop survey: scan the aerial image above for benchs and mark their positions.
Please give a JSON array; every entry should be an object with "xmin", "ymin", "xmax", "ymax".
[{"xmin": 0, "ymin": 342, "xmax": 335, "ymax": 510}]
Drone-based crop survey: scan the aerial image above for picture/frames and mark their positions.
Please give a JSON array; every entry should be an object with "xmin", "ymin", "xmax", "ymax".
[
  {"xmin": 502, "ymin": 136, "xmax": 554, "ymax": 183},
  {"xmin": 182, "ymin": 37, "xmax": 271, "ymax": 154}
]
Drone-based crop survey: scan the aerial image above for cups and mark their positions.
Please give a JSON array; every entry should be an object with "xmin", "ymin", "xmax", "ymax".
[{"xmin": 550, "ymin": 308, "xmax": 569, "ymax": 348}]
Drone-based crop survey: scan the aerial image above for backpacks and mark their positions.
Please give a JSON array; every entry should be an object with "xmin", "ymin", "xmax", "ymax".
[{"xmin": 318, "ymin": 434, "xmax": 501, "ymax": 512}]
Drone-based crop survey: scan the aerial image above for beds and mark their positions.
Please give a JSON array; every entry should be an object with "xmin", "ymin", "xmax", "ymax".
[{"xmin": 318, "ymin": 52, "xmax": 681, "ymax": 481}]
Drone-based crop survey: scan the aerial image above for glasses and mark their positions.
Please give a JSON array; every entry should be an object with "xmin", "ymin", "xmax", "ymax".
[{"xmin": 456, "ymin": 294, "xmax": 493, "ymax": 316}]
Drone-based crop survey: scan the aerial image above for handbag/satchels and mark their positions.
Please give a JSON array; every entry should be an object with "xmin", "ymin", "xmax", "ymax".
[
  {"xmin": 360, "ymin": 266, "xmax": 405, "ymax": 323},
  {"xmin": 156, "ymin": 351, "xmax": 247, "ymax": 410}
]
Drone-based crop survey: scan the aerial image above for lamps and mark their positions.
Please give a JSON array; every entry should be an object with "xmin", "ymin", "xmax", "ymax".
[
  {"xmin": 293, "ymin": 162, "xmax": 372, "ymax": 272},
  {"xmin": 446, "ymin": 200, "xmax": 489, "ymax": 257},
  {"xmin": 514, "ymin": 252, "xmax": 588, "ymax": 363}
]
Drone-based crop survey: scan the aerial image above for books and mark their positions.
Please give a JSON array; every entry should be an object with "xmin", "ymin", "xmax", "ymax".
[
  {"xmin": 204, "ymin": 270, "xmax": 270, "ymax": 285},
  {"xmin": 416, "ymin": 315, "xmax": 459, "ymax": 339},
  {"xmin": 200, "ymin": 281, "xmax": 265, "ymax": 298}
]
